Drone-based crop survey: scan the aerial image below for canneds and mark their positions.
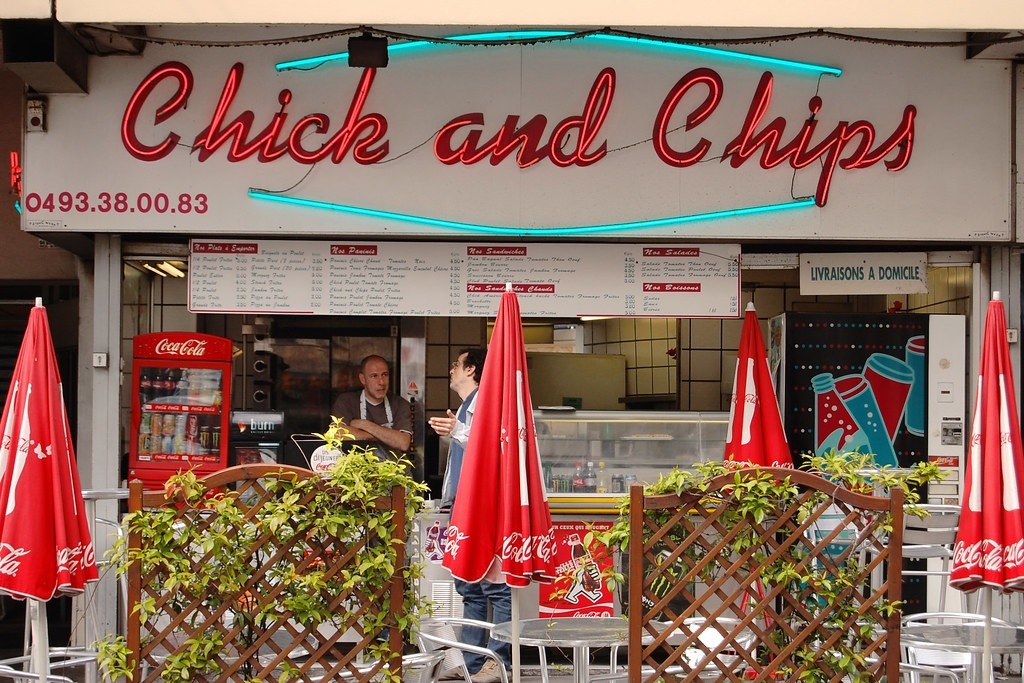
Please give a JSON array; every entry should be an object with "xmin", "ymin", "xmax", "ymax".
[
  {"xmin": 139, "ymin": 414, "xmax": 220, "ymax": 455},
  {"xmin": 552, "ymin": 475, "xmax": 561, "ymax": 493},
  {"xmin": 625, "ymin": 475, "xmax": 637, "ymax": 493},
  {"xmin": 612, "ymin": 474, "xmax": 623, "ymax": 492},
  {"xmin": 561, "ymin": 474, "xmax": 571, "ymax": 493}
]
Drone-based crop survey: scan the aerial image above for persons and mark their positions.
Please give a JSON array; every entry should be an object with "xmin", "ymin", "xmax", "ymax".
[
  {"xmin": 428, "ymin": 348, "xmax": 516, "ymax": 683},
  {"xmin": 327, "ymin": 356, "xmax": 416, "ymax": 649}
]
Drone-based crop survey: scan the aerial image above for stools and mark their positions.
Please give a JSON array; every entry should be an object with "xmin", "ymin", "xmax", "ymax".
[
  {"xmin": 846, "ymin": 504, "xmax": 968, "ymax": 651},
  {"xmin": 23, "ymin": 488, "xmax": 129, "ymax": 683}
]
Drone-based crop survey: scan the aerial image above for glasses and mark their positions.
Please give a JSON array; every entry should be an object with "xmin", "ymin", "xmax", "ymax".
[{"xmin": 452, "ymin": 361, "xmax": 468, "ymax": 368}]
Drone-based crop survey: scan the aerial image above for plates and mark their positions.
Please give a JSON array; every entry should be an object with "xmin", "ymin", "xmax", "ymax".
[{"xmin": 420, "ymin": 509, "xmax": 441, "ymax": 513}]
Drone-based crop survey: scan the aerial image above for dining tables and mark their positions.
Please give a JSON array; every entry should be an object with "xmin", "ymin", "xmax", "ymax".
[
  {"xmin": 869, "ymin": 625, "xmax": 1024, "ymax": 683},
  {"xmin": 140, "ymin": 628, "xmax": 320, "ymax": 683},
  {"xmin": 489, "ymin": 616, "xmax": 675, "ymax": 683}
]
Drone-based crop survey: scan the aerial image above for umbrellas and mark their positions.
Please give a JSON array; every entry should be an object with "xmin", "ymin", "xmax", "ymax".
[
  {"xmin": 0, "ymin": 297, "xmax": 100, "ymax": 683},
  {"xmin": 949, "ymin": 298, "xmax": 1024, "ymax": 683},
  {"xmin": 443, "ymin": 284, "xmax": 558, "ymax": 683},
  {"xmin": 717, "ymin": 305, "xmax": 796, "ymax": 662}
]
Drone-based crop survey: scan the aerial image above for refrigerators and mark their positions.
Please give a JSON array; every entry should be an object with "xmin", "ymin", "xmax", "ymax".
[{"xmin": 131, "ymin": 331, "xmax": 232, "ymax": 491}]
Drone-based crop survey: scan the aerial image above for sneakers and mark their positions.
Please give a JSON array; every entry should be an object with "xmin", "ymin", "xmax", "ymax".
[
  {"xmin": 438, "ymin": 666, "xmax": 465, "ymax": 680},
  {"xmin": 470, "ymin": 659, "xmax": 512, "ymax": 682}
]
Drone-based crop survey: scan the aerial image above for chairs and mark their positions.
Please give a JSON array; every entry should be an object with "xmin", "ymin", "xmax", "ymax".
[
  {"xmin": 412, "ymin": 616, "xmax": 549, "ymax": 683},
  {"xmin": 855, "ymin": 654, "xmax": 961, "ymax": 683},
  {"xmin": 588, "ymin": 616, "xmax": 756, "ymax": 682},
  {"xmin": 900, "ymin": 611, "xmax": 1012, "ymax": 683}
]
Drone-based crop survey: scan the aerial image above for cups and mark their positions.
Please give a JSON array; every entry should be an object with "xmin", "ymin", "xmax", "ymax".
[{"xmin": 422, "ymin": 500, "xmax": 435, "ymax": 509}]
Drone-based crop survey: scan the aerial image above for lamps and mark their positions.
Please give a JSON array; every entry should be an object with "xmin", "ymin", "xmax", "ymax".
[{"xmin": 348, "ymin": 30, "xmax": 389, "ymax": 68}]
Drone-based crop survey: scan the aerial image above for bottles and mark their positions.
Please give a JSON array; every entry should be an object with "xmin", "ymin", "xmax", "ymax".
[
  {"xmin": 585, "ymin": 461, "xmax": 597, "ymax": 493},
  {"xmin": 569, "ymin": 534, "xmax": 601, "ymax": 592},
  {"xmin": 426, "ymin": 521, "xmax": 440, "ymax": 552},
  {"xmin": 572, "ymin": 461, "xmax": 585, "ymax": 494},
  {"xmin": 544, "ymin": 462, "xmax": 554, "ymax": 492},
  {"xmin": 602, "ymin": 423, "xmax": 615, "ymax": 458},
  {"xmin": 597, "ymin": 461, "xmax": 608, "ymax": 493},
  {"xmin": 141, "ymin": 368, "xmax": 222, "ymax": 406}
]
